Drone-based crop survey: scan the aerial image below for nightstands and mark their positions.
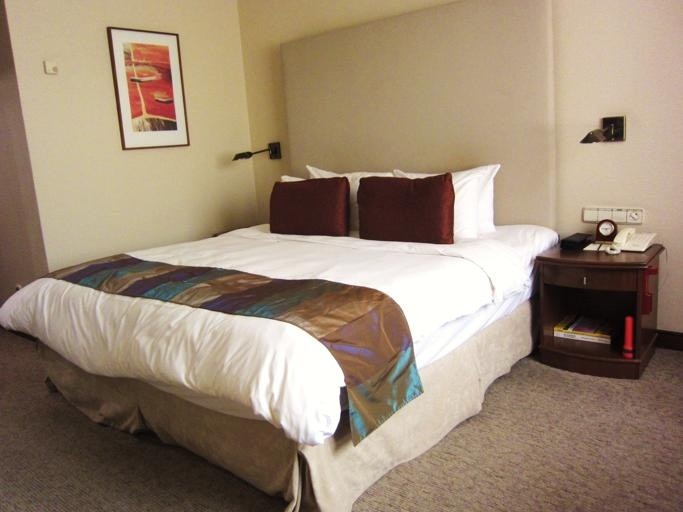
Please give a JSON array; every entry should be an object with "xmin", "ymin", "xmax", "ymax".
[{"xmin": 535, "ymin": 240, "xmax": 665, "ymax": 379}]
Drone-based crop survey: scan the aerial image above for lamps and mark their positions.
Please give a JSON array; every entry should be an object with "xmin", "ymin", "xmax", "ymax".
[
  {"xmin": 580, "ymin": 116, "xmax": 626, "ymax": 143},
  {"xmin": 232, "ymin": 142, "xmax": 282, "ymax": 161}
]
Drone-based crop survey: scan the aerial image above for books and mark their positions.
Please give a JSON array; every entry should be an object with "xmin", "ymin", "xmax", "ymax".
[
  {"xmin": 553, "ymin": 330, "xmax": 612, "ymax": 346},
  {"xmin": 553, "ymin": 313, "xmax": 616, "ymax": 339}
]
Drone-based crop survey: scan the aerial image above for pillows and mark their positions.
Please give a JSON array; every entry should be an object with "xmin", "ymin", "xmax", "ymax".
[
  {"xmin": 305, "ymin": 163, "xmax": 394, "ymax": 230},
  {"xmin": 357, "ymin": 171, "xmax": 455, "ymax": 244},
  {"xmin": 453, "ymin": 181, "xmax": 482, "ymax": 240},
  {"xmin": 269, "ymin": 176, "xmax": 350, "ymax": 237},
  {"xmin": 280, "ymin": 174, "xmax": 304, "ymax": 183},
  {"xmin": 394, "ymin": 163, "xmax": 502, "ymax": 235}
]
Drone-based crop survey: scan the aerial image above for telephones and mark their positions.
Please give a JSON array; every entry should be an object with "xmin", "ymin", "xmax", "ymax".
[{"xmin": 612, "ymin": 228, "xmax": 658, "ymax": 252}]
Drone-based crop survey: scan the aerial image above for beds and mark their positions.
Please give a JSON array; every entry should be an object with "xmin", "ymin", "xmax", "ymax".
[{"xmin": 0, "ymin": 223, "xmax": 560, "ymax": 512}]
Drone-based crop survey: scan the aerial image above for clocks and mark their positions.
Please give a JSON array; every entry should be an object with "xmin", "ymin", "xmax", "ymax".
[{"xmin": 596, "ymin": 219, "xmax": 618, "ymax": 242}]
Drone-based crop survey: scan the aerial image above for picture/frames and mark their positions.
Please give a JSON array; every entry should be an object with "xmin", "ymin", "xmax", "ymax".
[{"xmin": 107, "ymin": 27, "xmax": 190, "ymax": 150}]
